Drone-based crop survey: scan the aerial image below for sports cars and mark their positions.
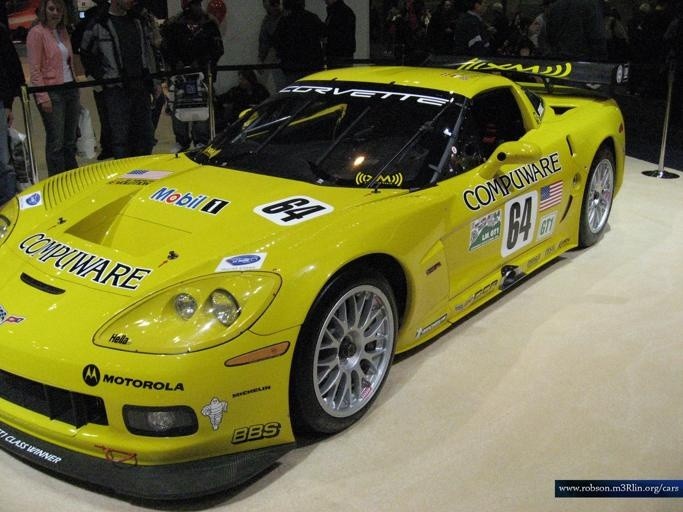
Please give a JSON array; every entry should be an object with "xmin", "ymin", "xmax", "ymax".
[{"xmin": 0, "ymin": 49, "xmax": 630, "ymax": 507}]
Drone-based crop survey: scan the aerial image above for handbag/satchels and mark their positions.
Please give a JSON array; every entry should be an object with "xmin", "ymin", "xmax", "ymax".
[
  {"xmin": 607, "ymin": 37, "xmax": 628, "ymax": 63},
  {"xmin": 76, "ymin": 105, "xmax": 98, "ymax": 160},
  {"xmin": 7, "ymin": 127, "xmax": 38, "ymax": 191},
  {"xmin": 169, "ymin": 72, "xmax": 209, "ymax": 122}
]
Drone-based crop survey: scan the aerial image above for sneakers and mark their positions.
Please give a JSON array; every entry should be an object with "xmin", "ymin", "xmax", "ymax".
[{"xmin": 171, "ymin": 143, "xmax": 189, "ymax": 153}]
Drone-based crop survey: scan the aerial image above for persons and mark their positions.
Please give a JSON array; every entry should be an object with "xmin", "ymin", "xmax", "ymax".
[{"xmin": 0, "ymin": 1, "xmax": 682, "ymax": 210}]
